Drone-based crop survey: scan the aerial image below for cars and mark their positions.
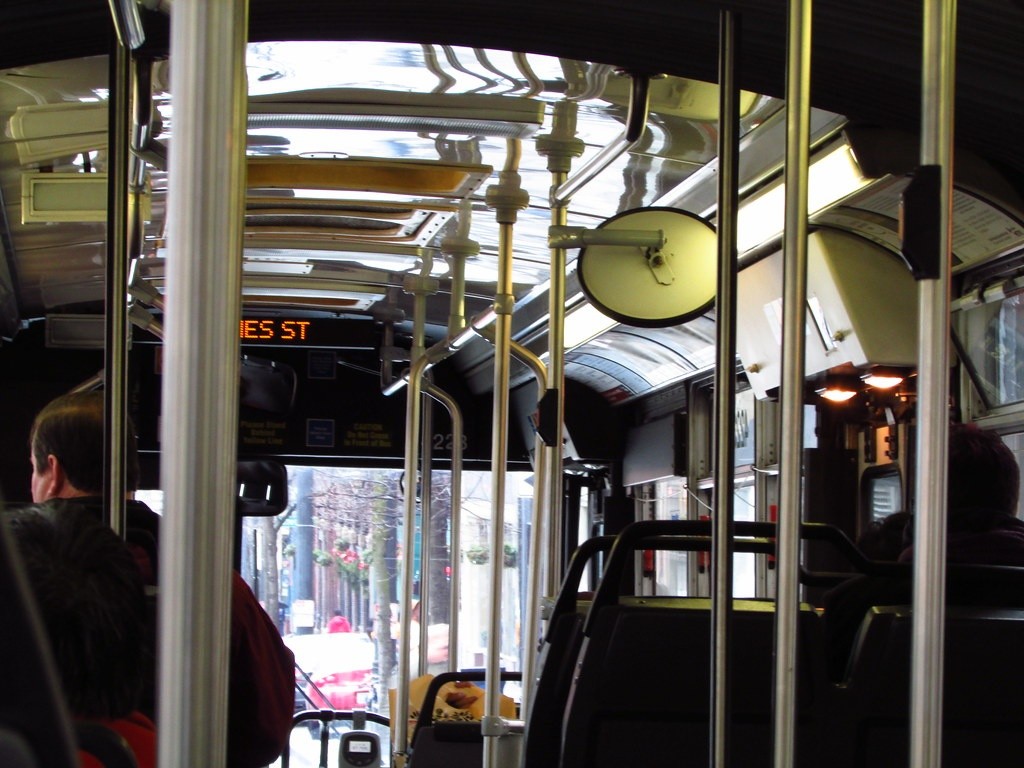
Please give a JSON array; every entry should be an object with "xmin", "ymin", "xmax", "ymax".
[{"xmin": 281, "ymin": 623, "xmax": 449, "ymax": 741}]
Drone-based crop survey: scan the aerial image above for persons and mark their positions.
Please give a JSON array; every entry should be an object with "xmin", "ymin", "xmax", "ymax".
[
  {"xmin": 834, "ymin": 424, "xmax": 1024, "ymax": 608},
  {"xmin": 0, "ymin": 387, "xmax": 294, "ymax": 768},
  {"xmin": 327, "ymin": 610, "xmax": 352, "ymax": 633}
]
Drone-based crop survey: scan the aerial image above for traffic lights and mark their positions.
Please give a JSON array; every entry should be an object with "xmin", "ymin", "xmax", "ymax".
[{"xmin": 446, "ymin": 553, "xmax": 451, "ymax": 578}]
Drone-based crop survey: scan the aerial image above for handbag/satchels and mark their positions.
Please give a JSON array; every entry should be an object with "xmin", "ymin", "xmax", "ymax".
[{"xmin": 388, "ymin": 673, "xmax": 516, "ymax": 768}]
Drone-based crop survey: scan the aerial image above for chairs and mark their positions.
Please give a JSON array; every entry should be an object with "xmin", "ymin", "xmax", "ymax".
[{"xmin": 407, "ymin": 514, "xmax": 1024, "ymax": 768}]
